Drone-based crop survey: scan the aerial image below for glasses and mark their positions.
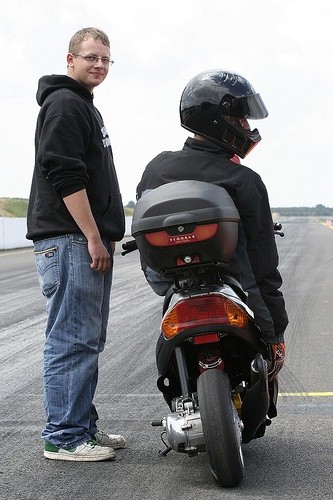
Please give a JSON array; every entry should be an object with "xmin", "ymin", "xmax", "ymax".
[{"xmin": 74, "ymin": 52, "xmax": 115, "ymax": 68}]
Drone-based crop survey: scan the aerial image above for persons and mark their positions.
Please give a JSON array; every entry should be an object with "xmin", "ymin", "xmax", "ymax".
[
  {"xmin": 26, "ymin": 27, "xmax": 128, "ymax": 463},
  {"xmin": 133, "ymin": 67, "xmax": 289, "ymax": 381}
]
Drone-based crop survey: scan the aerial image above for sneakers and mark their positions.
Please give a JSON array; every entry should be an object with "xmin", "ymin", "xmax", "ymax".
[
  {"xmin": 44, "ymin": 437, "xmax": 116, "ymax": 462},
  {"xmin": 91, "ymin": 429, "xmax": 127, "ymax": 449}
]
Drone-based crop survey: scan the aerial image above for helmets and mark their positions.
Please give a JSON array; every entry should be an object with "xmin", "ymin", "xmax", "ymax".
[{"xmin": 176, "ymin": 70, "xmax": 263, "ymax": 161}]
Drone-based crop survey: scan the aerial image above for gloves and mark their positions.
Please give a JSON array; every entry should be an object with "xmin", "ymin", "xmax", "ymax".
[{"xmin": 268, "ymin": 340, "xmax": 286, "ymax": 366}]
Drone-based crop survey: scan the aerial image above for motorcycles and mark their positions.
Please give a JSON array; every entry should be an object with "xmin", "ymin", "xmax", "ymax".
[{"xmin": 122, "ymin": 221, "xmax": 286, "ymax": 488}]
